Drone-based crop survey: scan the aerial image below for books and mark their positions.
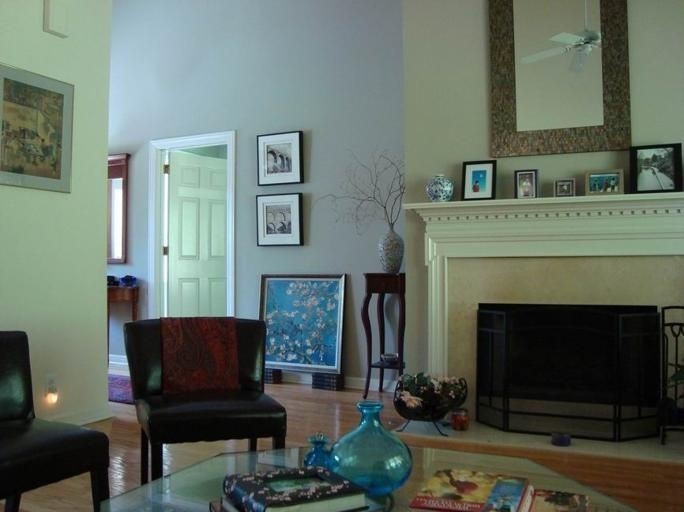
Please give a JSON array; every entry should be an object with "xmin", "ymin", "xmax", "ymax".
[
  {"xmin": 311, "ymin": 372, "xmax": 346, "ymax": 391},
  {"xmin": 208, "ymin": 500, "xmax": 226, "ymax": 512},
  {"xmin": 220, "ymin": 494, "xmax": 238, "ymax": 512},
  {"xmin": 406, "ymin": 468, "xmax": 535, "ymax": 512},
  {"xmin": 529, "ymin": 485, "xmax": 589, "ymax": 512},
  {"xmin": 222, "ymin": 464, "xmax": 371, "ymax": 512},
  {"xmin": 263, "ymin": 367, "xmax": 283, "ymax": 385}
]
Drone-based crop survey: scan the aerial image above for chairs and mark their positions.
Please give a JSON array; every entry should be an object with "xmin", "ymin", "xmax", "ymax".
[
  {"xmin": 0, "ymin": 330, "xmax": 110, "ymax": 512},
  {"xmin": 122, "ymin": 316, "xmax": 287, "ymax": 487}
]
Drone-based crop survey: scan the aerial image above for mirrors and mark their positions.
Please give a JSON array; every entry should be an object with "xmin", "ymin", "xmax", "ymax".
[
  {"xmin": 488, "ymin": 1, "xmax": 633, "ymax": 158},
  {"xmin": 108, "ymin": 153, "xmax": 131, "ymax": 265}
]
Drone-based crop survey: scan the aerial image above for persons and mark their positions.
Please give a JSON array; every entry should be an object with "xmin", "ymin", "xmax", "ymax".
[
  {"xmin": 610, "ymin": 178, "xmax": 616, "ymax": 192},
  {"xmin": 562, "ymin": 185, "xmax": 567, "ymax": 193},
  {"xmin": 522, "ymin": 177, "xmax": 532, "ymax": 196},
  {"xmin": 0, "ymin": 119, "xmax": 60, "ymax": 177},
  {"xmin": 603, "ymin": 179, "xmax": 608, "ymax": 192},
  {"xmin": 591, "ymin": 178, "xmax": 601, "ymax": 192},
  {"xmin": 472, "ymin": 180, "xmax": 481, "ymax": 193}
]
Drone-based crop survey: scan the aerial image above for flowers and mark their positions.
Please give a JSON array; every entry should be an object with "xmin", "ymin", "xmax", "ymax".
[{"xmin": 399, "ymin": 371, "xmax": 466, "ymax": 410}]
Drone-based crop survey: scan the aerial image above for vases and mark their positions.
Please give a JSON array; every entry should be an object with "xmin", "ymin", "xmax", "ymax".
[
  {"xmin": 424, "ymin": 173, "xmax": 454, "ymax": 202},
  {"xmin": 391, "ymin": 378, "xmax": 468, "ymax": 436},
  {"xmin": 330, "ymin": 402, "xmax": 413, "ymax": 497},
  {"xmin": 377, "ymin": 223, "xmax": 404, "ymax": 275}
]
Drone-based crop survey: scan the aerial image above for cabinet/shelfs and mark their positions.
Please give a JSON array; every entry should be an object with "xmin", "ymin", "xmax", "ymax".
[{"xmin": 361, "ymin": 272, "xmax": 406, "ymax": 399}]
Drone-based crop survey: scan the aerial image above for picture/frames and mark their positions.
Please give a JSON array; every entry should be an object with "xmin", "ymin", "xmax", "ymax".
[
  {"xmin": 259, "ymin": 274, "xmax": 348, "ymax": 376},
  {"xmin": 553, "ymin": 178, "xmax": 576, "ymax": 196},
  {"xmin": 461, "ymin": 160, "xmax": 496, "ymax": 201},
  {"xmin": 514, "ymin": 169, "xmax": 537, "ymax": 199},
  {"xmin": 585, "ymin": 168, "xmax": 624, "ymax": 196},
  {"xmin": 256, "ymin": 192, "xmax": 304, "ymax": 246},
  {"xmin": 0, "ymin": 62, "xmax": 75, "ymax": 194},
  {"xmin": 256, "ymin": 130, "xmax": 305, "ymax": 186},
  {"xmin": 629, "ymin": 142, "xmax": 681, "ymax": 194}
]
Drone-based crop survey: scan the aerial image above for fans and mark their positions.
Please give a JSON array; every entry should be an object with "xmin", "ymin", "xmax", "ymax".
[{"xmin": 519, "ymin": 0, "xmax": 602, "ymax": 67}]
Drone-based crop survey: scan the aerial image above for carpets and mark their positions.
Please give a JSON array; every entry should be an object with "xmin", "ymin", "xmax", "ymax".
[{"xmin": 109, "ymin": 373, "xmax": 135, "ymax": 405}]
